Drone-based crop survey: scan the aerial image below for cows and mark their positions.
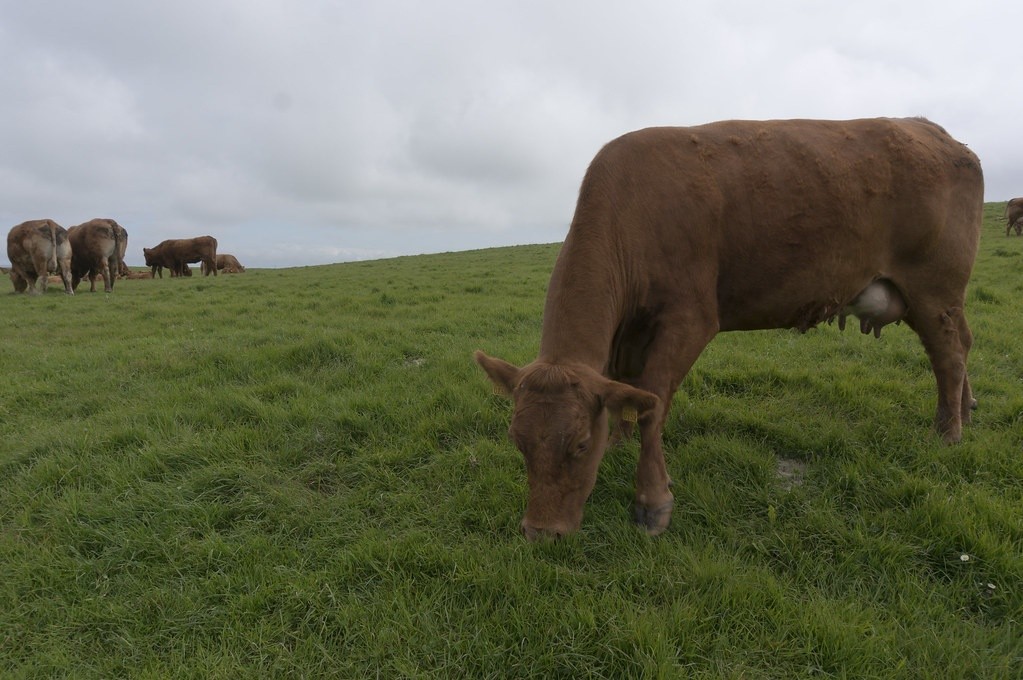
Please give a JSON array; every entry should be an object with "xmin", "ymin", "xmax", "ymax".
[
  {"xmin": 1004, "ymin": 197, "xmax": 1023, "ymax": 237},
  {"xmin": 1, "ymin": 217, "xmax": 245, "ymax": 298},
  {"xmin": 473, "ymin": 116, "xmax": 985, "ymax": 542}
]
